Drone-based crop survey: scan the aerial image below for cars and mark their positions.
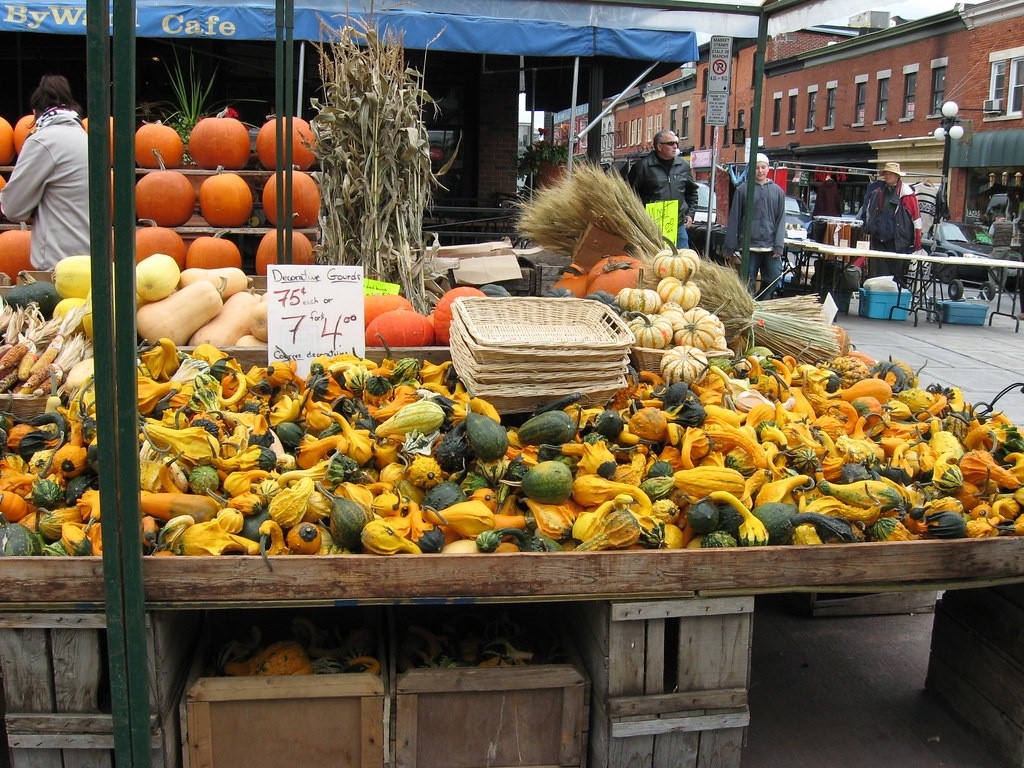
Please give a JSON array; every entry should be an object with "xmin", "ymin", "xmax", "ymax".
[
  {"xmin": 921, "ymin": 222, "xmax": 1024, "ymax": 286},
  {"xmin": 783, "ymin": 195, "xmax": 814, "ymax": 241},
  {"xmin": 692, "ymin": 181, "xmax": 717, "ymax": 224}
]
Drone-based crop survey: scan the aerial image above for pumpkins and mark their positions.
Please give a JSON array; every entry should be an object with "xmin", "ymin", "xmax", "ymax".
[
  {"xmin": 498, "ymin": 460, "xmax": 573, "ymax": 504},
  {"xmin": 0, "ymin": 511, "xmax": 43, "ymax": 556},
  {"xmin": 517, "ymin": 409, "xmax": 576, "ymax": 448},
  {"xmin": 0, "ymin": 110, "xmax": 515, "ymax": 351},
  {"xmin": 464, "ymin": 402, "xmax": 509, "ymax": 459},
  {"xmin": 549, "ymin": 235, "xmax": 732, "ymax": 386},
  {"xmin": 313, "ymin": 480, "xmax": 369, "ymax": 549},
  {"xmin": 629, "ymin": 398, "xmax": 669, "ymax": 442}
]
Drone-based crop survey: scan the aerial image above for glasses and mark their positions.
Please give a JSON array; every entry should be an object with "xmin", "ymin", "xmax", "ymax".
[{"xmin": 660, "ymin": 141, "xmax": 678, "ymax": 146}]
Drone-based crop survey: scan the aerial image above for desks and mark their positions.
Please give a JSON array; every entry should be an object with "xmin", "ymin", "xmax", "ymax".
[
  {"xmin": 685, "ymin": 222, "xmax": 835, "ymax": 298},
  {"xmin": 803, "ymin": 247, "xmax": 942, "ymax": 328},
  {"xmin": 909, "ymin": 254, "xmax": 1024, "ymax": 333}
]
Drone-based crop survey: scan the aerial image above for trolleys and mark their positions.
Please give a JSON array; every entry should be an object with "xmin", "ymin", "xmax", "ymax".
[{"xmin": 911, "ymin": 278, "xmax": 995, "ymax": 305}]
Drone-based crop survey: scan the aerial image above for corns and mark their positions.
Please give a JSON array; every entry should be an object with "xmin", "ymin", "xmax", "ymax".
[{"xmin": 0, "ymin": 342, "xmax": 65, "ymax": 397}]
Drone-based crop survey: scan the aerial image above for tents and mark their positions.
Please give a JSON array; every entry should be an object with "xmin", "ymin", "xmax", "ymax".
[{"xmin": 1, "ymin": 0, "xmax": 701, "ymax": 67}]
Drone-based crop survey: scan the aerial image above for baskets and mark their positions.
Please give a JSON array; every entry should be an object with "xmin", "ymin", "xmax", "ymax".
[
  {"xmin": 449, "ymin": 297, "xmax": 635, "ymax": 414},
  {"xmin": 630, "ymin": 343, "xmax": 734, "ymax": 385}
]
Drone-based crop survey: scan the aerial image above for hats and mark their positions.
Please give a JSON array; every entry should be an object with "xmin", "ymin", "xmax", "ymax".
[
  {"xmin": 1015, "ymin": 172, "xmax": 1022, "ymax": 176},
  {"xmin": 757, "ymin": 153, "xmax": 769, "ymax": 165},
  {"xmin": 879, "ymin": 163, "xmax": 906, "ymax": 176},
  {"xmin": 989, "ymin": 173, "xmax": 996, "ymax": 177},
  {"xmin": 993, "ymin": 213, "xmax": 1003, "ymax": 221},
  {"xmin": 1002, "ymin": 172, "xmax": 1009, "ymax": 175}
]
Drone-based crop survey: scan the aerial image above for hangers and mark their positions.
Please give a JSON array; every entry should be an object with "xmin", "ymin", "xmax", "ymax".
[
  {"xmin": 908, "ymin": 174, "xmax": 949, "ymax": 202},
  {"xmin": 814, "ymin": 215, "xmax": 864, "ymax": 231}
]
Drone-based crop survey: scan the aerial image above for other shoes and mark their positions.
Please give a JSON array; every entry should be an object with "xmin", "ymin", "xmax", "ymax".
[{"xmin": 995, "ymin": 285, "xmax": 1004, "ymax": 292}]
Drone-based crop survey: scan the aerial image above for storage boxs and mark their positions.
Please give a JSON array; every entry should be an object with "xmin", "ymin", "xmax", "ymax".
[
  {"xmin": 936, "ymin": 301, "xmax": 989, "ymax": 326},
  {"xmin": 179, "ymin": 610, "xmax": 390, "ymax": 768},
  {"xmin": 391, "ymin": 608, "xmax": 592, "ymax": 768},
  {"xmin": 858, "ymin": 288, "xmax": 911, "ymax": 321}
]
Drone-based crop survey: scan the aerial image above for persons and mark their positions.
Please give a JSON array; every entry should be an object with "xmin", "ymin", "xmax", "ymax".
[
  {"xmin": 989, "ymin": 209, "xmax": 1024, "ymax": 320},
  {"xmin": 856, "ymin": 163, "xmax": 921, "ymax": 289},
  {"xmin": 0, "ymin": 74, "xmax": 90, "ymax": 272},
  {"xmin": 721, "ymin": 154, "xmax": 786, "ymax": 301},
  {"xmin": 628, "ymin": 131, "xmax": 699, "ymax": 249}
]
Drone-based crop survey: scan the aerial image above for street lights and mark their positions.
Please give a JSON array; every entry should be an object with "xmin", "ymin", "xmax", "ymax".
[{"xmin": 933, "ymin": 101, "xmax": 964, "ymax": 222}]
